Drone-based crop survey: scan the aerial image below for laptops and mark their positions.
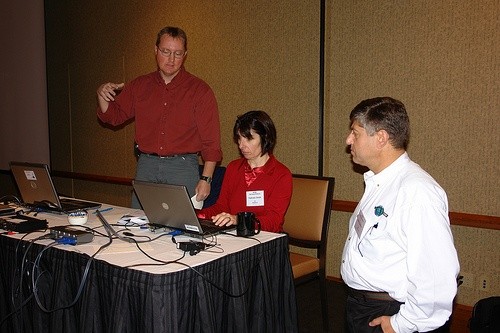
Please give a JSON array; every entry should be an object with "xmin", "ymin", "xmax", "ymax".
[
  {"xmin": 11, "ymin": 162, "xmax": 102, "ymax": 214},
  {"xmin": 133, "ymin": 180, "xmax": 237, "ymax": 239}
]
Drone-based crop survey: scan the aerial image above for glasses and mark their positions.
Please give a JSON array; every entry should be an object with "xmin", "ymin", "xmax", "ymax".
[{"xmin": 157, "ymin": 46, "xmax": 186, "ymax": 59}]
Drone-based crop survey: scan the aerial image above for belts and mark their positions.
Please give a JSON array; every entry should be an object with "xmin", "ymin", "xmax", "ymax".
[
  {"xmin": 347, "ymin": 286, "xmax": 396, "ymax": 301},
  {"xmin": 141, "ymin": 152, "xmax": 197, "ymax": 159}
]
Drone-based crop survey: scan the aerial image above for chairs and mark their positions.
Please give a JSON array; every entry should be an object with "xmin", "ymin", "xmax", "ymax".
[{"xmin": 282, "ymin": 174, "xmax": 335, "ymax": 333}]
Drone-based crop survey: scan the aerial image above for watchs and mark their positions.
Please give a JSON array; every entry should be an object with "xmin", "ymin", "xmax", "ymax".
[{"xmin": 200, "ymin": 176, "xmax": 213, "ymax": 184}]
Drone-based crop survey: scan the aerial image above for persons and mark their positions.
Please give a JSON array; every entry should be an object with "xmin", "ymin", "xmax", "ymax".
[
  {"xmin": 95, "ymin": 26, "xmax": 222, "ymax": 208},
  {"xmin": 340, "ymin": 97, "xmax": 460, "ymax": 333},
  {"xmin": 197, "ymin": 110, "xmax": 293, "ymax": 232}
]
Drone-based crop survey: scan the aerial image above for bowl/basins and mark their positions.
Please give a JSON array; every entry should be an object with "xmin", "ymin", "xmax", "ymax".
[{"xmin": 68, "ymin": 212, "xmax": 88, "ymax": 225}]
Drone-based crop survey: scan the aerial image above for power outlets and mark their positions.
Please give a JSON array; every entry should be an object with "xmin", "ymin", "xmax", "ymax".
[
  {"xmin": 455, "ymin": 273, "xmax": 474, "ymax": 288},
  {"xmin": 480, "ymin": 276, "xmax": 490, "ymax": 292}
]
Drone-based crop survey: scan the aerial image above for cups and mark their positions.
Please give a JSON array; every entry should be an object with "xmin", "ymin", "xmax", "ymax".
[{"xmin": 237, "ymin": 211, "xmax": 261, "ymax": 237}]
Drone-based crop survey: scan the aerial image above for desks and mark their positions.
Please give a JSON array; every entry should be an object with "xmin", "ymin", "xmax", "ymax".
[{"xmin": 0, "ymin": 195, "xmax": 299, "ymax": 333}]
